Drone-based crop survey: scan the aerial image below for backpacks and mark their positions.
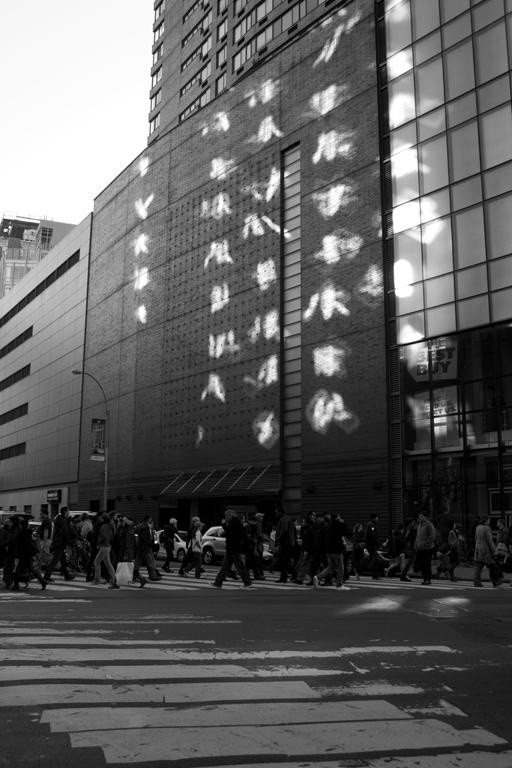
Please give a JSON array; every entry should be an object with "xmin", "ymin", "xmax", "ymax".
[{"xmin": 159, "ymin": 529, "xmax": 169, "ymax": 543}]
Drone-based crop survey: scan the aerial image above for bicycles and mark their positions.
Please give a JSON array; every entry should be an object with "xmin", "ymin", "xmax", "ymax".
[{"xmin": 48, "ymin": 536, "xmax": 97, "ymax": 575}]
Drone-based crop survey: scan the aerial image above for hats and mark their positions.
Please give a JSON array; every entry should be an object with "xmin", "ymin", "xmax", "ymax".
[
  {"xmin": 255, "ymin": 513, "xmax": 264, "ymax": 519},
  {"xmin": 170, "ymin": 519, "xmax": 176, "ymax": 523},
  {"xmin": 192, "ymin": 517, "xmax": 200, "ymax": 522},
  {"xmin": 102, "ymin": 513, "xmax": 110, "ymax": 522},
  {"xmin": 195, "ymin": 522, "xmax": 204, "ymax": 527}
]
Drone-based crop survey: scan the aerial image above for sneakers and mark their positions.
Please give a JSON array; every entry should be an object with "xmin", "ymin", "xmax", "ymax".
[
  {"xmin": 473, "ymin": 581, "xmax": 482, "ymax": 587},
  {"xmin": 385, "ymin": 568, "xmax": 389, "ymax": 576},
  {"xmin": 494, "ymin": 580, "xmax": 502, "ymax": 586},
  {"xmin": 275, "ymin": 575, "xmax": 351, "ymax": 590},
  {"xmin": 372, "ymin": 576, "xmax": 384, "ymax": 581},
  {"xmin": 209, "ymin": 574, "xmax": 266, "ymax": 590},
  {"xmin": 449, "ymin": 574, "xmax": 457, "ymax": 581},
  {"xmin": 5, "ymin": 573, "xmax": 75, "ymax": 590},
  {"xmin": 422, "ymin": 579, "xmax": 431, "ymax": 584},
  {"xmin": 162, "ymin": 565, "xmax": 206, "ymax": 579},
  {"xmin": 86, "ymin": 572, "xmax": 164, "ymax": 588},
  {"xmin": 400, "ymin": 575, "xmax": 412, "ymax": 582}
]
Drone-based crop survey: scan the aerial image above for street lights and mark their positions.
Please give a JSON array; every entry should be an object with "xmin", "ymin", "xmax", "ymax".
[{"xmin": 72, "ymin": 370, "xmax": 110, "ymax": 513}]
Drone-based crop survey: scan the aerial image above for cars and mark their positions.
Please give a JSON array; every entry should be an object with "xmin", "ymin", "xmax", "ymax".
[{"xmin": 153, "ymin": 520, "xmax": 401, "ymax": 577}]
